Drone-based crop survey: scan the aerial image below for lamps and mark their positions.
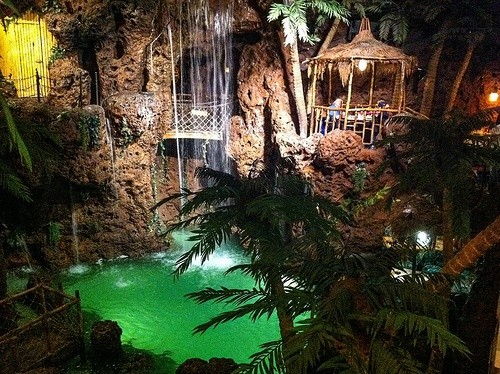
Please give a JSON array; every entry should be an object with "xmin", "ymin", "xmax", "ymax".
[
  {"xmin": 489, "ymin": 87, "xmax": 499, "ymax": 102},
  {"xmin": 359, "ymin": 59, "xmax": 367, "ymax": 72}
]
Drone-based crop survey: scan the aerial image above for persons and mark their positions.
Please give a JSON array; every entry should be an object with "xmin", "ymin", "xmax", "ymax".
[
  {"xmin": 376, "ymin": 99, "xmax": 392, "ymax": 132},
  {"xmin": 320, "ymin": 93, "xmax": 347, "ymax": 137}
]
